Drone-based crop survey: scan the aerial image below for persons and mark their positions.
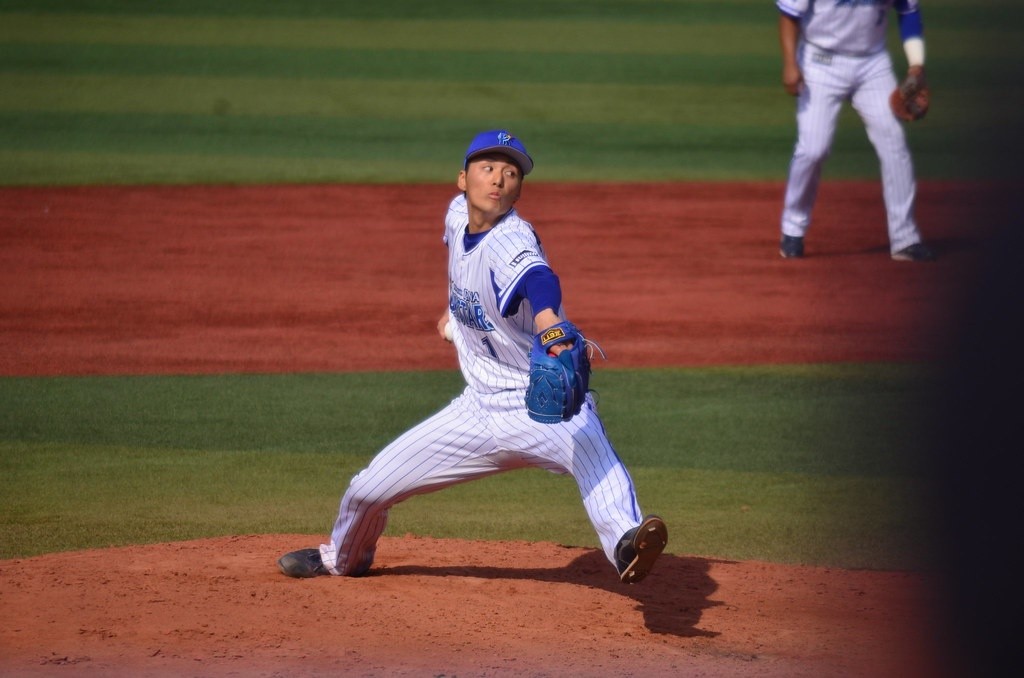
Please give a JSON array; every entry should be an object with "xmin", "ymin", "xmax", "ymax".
[
  {"xmin": 775, "ymin": 0, "xmax": 938, "ymax": 263},
  {"xmin": 278, "ymin": 130, "xmax": 668, "ymax": 583}
]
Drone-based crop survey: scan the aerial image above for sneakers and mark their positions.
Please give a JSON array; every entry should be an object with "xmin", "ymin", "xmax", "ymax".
[
  {"xmin": 615, "ymin": 514, "xmax": 667, "ymax": 582},
  {"xmin": 275, "ymin": 550, "xmax": 328, "ymax": 576}
]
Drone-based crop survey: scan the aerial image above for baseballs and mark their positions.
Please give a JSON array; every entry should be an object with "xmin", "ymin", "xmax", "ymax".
[{"xmin": 444, "ymin": 319, "xmax": 456, "ymax": 342}]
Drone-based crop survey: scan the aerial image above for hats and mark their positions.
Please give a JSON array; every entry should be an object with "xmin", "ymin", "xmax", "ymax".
[{"xmin": 464, "ymin": 129, "xmax": 533, "ymax": 177}]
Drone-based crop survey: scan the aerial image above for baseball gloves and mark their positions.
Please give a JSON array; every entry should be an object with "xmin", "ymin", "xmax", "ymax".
[
  {"xmin": 525, "ymin": 319, "xmax": 591, "ymax": 425},
  {"xmin": 889, "ymin": 77, "xmax": 934, "ymax": 124}
]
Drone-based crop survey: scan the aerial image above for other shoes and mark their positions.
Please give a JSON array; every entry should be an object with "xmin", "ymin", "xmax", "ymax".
[
  {"xmin": 781, "ymin": 233, "xmax": 801, "ymax": 257},
  {"xmin": 893, "ymin": 243, "xmax": 939, "ymax": 262}
]
